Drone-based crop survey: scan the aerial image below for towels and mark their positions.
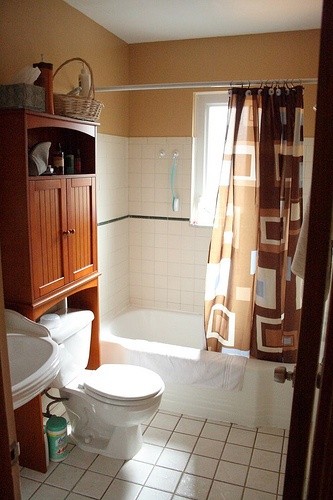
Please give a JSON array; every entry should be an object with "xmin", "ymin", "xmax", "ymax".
[
  {"xmin": 290, "ymin": 188, "xmax": 311, "ymax": 280},
  {"xmin": 144, "ymin": 340, "xmax": 247, "ymax": 392}
]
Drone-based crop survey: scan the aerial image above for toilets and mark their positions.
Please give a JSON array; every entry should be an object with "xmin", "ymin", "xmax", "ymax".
[{"xmin": 37, "ymin": 307, "xmax": 165, "ymax": 461}]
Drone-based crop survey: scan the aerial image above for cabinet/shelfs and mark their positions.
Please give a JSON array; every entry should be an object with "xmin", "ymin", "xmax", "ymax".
[{"xmin": 0, "ymin": 107, "xmax": 101, "ymax": 473}]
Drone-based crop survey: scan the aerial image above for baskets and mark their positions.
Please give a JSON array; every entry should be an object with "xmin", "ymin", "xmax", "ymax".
[{"xmin": 53, "ymin": 58, "xmax": 104, "ymax": 122}]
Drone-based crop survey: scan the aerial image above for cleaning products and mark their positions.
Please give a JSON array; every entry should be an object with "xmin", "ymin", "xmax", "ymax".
[{"xmin": 45, "ymin": 417, "xmax": 68, "ymax": 460}]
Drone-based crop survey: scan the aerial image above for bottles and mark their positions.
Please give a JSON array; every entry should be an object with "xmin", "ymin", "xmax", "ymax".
[
  {"xmin": 53, "ymin": 141, "xmax": 64, "ymax": 175},
  {"xmin": 75, "ymin": 148, "xmax": 81, "ymax": 173},
  {"xmin": 65, "ymin": 143, "xmax": 74, "ymax": 174}
]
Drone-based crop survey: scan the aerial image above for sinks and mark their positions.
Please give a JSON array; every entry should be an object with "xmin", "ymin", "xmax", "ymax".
[{"xmin": 7, "ymin": 334, "xmax": 61, "ymax": 411}]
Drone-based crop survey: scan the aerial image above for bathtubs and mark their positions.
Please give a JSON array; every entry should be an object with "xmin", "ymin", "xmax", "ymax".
[{"xmin": 100, "ymin": 307, "xmax": 294, "ymax": 431}]
